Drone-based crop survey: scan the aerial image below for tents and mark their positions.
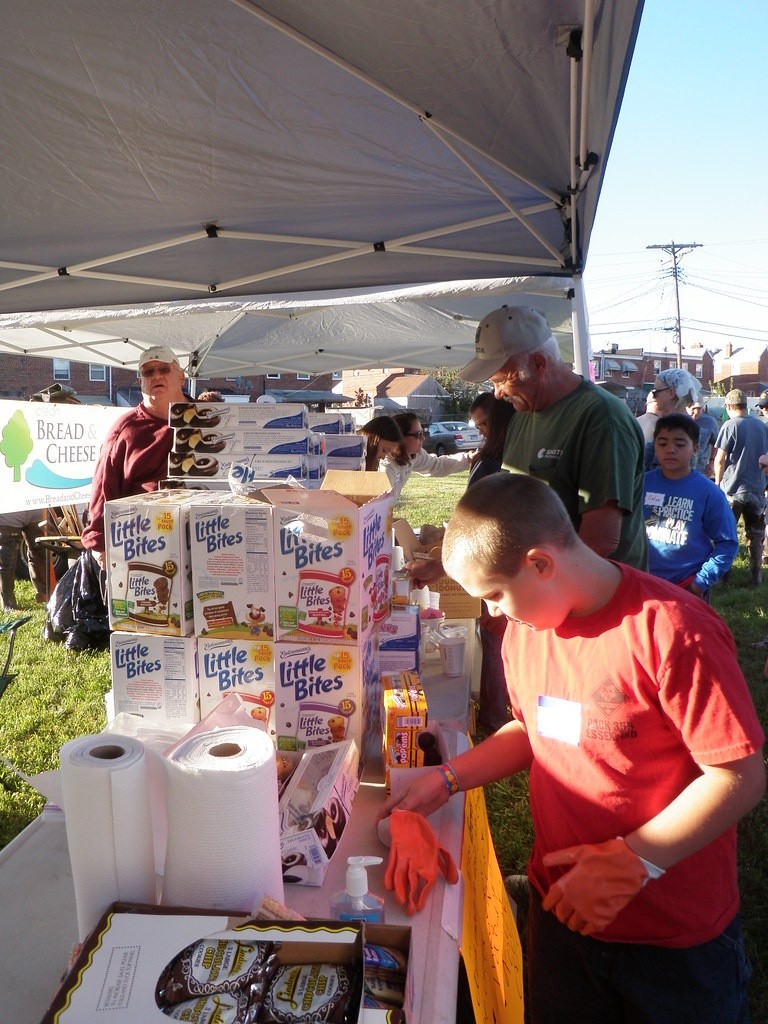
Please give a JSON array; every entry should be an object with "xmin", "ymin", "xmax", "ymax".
[{"xmin": 1, "ymin": 1, "xmax": 644, "ymax": 397}]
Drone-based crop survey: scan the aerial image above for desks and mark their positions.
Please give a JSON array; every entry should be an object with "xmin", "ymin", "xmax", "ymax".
[
  {"xmin": 421, "ymin": 617, "xmax": 476, "ymax": 730},
  {"xmin": 1, "ymin": 729, "xmax": 478, "ymax": 1024}
]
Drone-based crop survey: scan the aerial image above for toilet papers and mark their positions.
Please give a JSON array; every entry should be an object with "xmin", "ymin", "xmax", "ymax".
[
  {"xmin": 148, "ymin": 724, "xmax": 286, "ymax": 917},
  {"xmin": 0, "ymin": 733, "xmax": 158, "ymax": 948}
]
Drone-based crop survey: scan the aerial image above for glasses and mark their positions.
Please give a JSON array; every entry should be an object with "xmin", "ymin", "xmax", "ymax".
[
  {"xmin": 403, "ymin": 430, "xmax": 424, "ymax": 439},
  {"xmin": 475, "ymin": 419, "xmax": 488, "ymax": 430},
  {"xmin": 652, "ymin": 387, "xmax": 670, "ymax": 399},
  {"xmin": 759, "ymin": 405, "xmax": 768, "ymax": 409},
  {"xmin": 141, "ymin": 365, "xmax": 181, "ymax": 378}
]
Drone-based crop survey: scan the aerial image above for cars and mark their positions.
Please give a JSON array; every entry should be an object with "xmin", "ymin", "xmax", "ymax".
[{"xmin": 425, "ymin": 421, "xmax": 484, "ymax": 456}]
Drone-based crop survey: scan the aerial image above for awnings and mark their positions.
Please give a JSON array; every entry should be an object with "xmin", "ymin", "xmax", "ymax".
[
  {"xmin": 623, "ymin": 362, "xmax": 638, "ymax": 371},
  {"xmin": 606, "ymin": 361, "xmax": 621, "ymax": 371}
]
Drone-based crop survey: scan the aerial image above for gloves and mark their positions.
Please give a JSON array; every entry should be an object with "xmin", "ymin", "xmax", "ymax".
[
  {"xmin": 377, "ymin": 810, "xmax": 459, "ymax": 916},
  {"xmin": 542, "ymin": 836, "xmax": 666, "ymax": 937}
]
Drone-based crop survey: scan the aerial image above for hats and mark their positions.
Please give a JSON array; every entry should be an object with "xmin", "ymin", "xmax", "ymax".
[
  {"xmin": 454, "ymin": 304, "xmax": 552, "ymax": 383},
  {"xmin": 722, "ymin": 389, "xmax": 747, "ymax": 408},
  {"xmin": 691, "ymin": 394, "xmax": 704, "ymax": 408},
  {"xmin": 139, "ymin": 345, "xmax": 181, "ymax": 368},
  {"xmin": 755, "ymin": 389, "xmax": 768, "ymax": 407}
]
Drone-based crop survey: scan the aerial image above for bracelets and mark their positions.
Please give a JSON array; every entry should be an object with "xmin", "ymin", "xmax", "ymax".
[{"xmin": 436, "ymin": 762, "xmax": 460, "ymax": 797}]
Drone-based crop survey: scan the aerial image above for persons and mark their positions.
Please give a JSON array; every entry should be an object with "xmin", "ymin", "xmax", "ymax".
[
  {"xmin": 380, "ymin": 412, "xmax": 480, "ymax": 498},
  {"xmin": 198, "ymin": 391, "xmax": 225, "ymax": 402},
  {"xmin": 642, "ymin": 413, "xmax": 738, "ymax": 604},
  {"xmin": 356, "ymin": 415, "xmax": 404, "ymax": 471},
  {"xmin": 470, "ymin": 392, "xmax": 497, "ymax": 438},
  {"xmin": 402, "ymin": 305, "xmax": 646, "ymax": 732},
  {"xmin": 714, "ymin": 389, "xmax": 768, "ymax": 584},
  {"xmin": 377, "ymin": 472, "xmax": 768, "ymax": 1024},
  {"xmin": 81, "ymin": 346, "xmax": 209, "ymax": 572},
  {"xmin": 601, "ymin": 368, "xmax": 768, "ymax": 564},
  {"xmin": 0, "ymin": 509, "xmax": 46, "ymax": 610}
]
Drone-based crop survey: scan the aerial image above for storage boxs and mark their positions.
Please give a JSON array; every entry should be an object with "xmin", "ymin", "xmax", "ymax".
[
  {"xmin": 379, "ymin": 670, "xmax": 428, "ymax": 794},
  {"xmin": 275, "ymin": 640, "xmax": 380, "ymax": 757},
  {"xmin": 41, "ymin": 901, "xmax": 415, "ymax": 1024},
  {"xmin": 188, "ymin": 486, "xmax": 276, "ymax": 640},
  {"xmin": 110, "ymin": 630, "xmax": 201, "ymax": 726},
  {"xmin": 197, "ymin": 637, "xmax": 276, "ymax": 751},
  {"xmin": 243, "ymin": 469, "xmax": 397, "ymax": 643},
  {"xmin": 104, "ymin": 489, "xmax": 227, "ymax": 637},
  {"xmin": 157, "ymin": 401, "xmax": 369, "ymax": 492},
  {"xmin": 380, "ymin": 518, "xmax": 482, "ymax": 672}
]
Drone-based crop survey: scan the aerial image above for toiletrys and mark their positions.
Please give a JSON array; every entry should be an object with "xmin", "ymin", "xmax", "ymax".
[{"xmin": 328, "ymin": 855, "xmax": 386, "ymax": 923}]
